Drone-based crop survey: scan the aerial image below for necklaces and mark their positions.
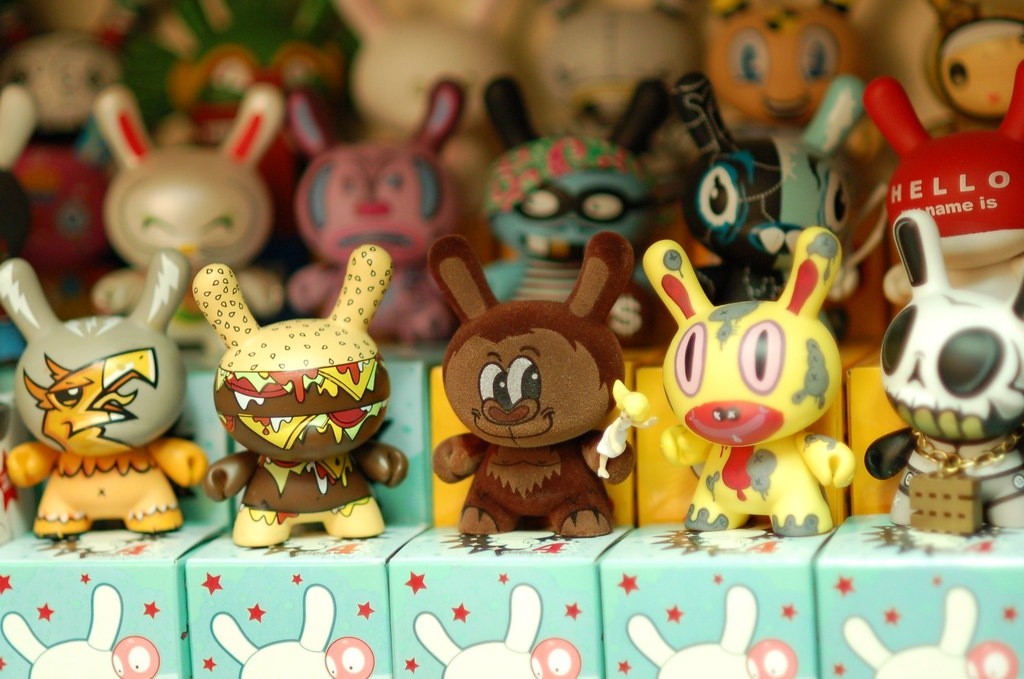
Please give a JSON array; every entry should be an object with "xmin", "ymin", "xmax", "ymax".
[{"xmin": 911, "ymin": 429, "xmax": 1024, "ymax": 536}]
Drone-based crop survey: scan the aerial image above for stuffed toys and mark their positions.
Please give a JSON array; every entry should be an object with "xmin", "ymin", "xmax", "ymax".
[{"xmin": 0, "ymin": 0, "xmax": 1024, "ymax": 548}]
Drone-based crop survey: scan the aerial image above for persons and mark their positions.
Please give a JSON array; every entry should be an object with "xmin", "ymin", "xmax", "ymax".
[{"xmin": 595, "ymin": 380, "xmax": 659, "ymax": 479}]
